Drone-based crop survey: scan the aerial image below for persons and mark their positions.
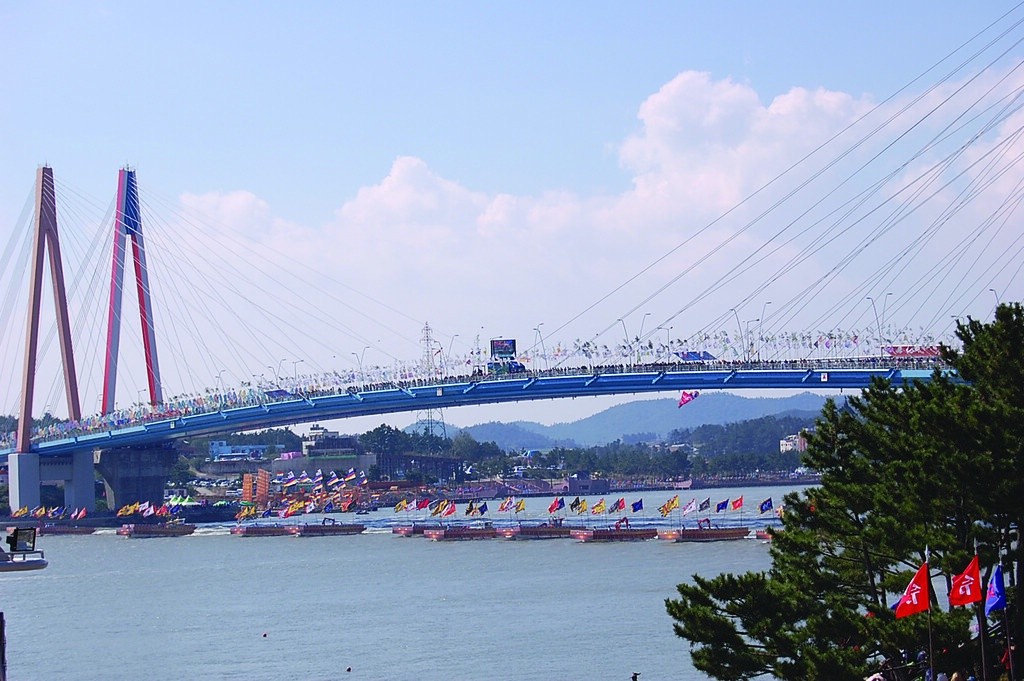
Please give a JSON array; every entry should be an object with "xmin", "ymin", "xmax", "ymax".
[
  {"xmin": 594, "ymin": 524, "xmax": 631, "ymax": 531},
  {"xmin": 861, "ymin": 640, "xmax": 1017, "ymax": 681},
  {"xmin": 305, "ymin": 523, "xmax": 308, "ymax": 528},
  {"xmin": 552, "ymin": 518, "xmax": 564, "ymax": 529},
  {"xmin": 276, "ymin": 523, "xmax": 278, "ymax": 528},
  {"xmin": 682, "ymin": 519, "xmax": 721, "ymax": 530},
  {"xmin": 0, "ymin": 358, "xmax": 954, "ymax": 496},
  {"xmin": 412, "ymin": 522, "xmax": 415, "ymax": 527},
  {"xmin": 75, "ymin": 526, "xmax": 78, "ymax": 529},
  {"xmin": 341, "ymin": 522, "xmax": 343, "ymax": 527},
  {"xmin": 255, "ymin": 523, "xmax": 259, "ymax": 528},
  {"xmin": 446, "ymin": 524, "xmax": 450, "ymax": 532}
]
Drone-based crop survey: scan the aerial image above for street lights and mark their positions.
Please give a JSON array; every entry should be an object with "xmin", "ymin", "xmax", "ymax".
[
  {"xmin": 881, "ymin": 292, "xmax": 894, "ymax": 336},
  {"xmin": 746, "ymin": 318, "xmax": 760, "ymax": 369},
  {"xmin": 657, "ymin": 326, "xmax": 673, "ymax": 365},
  {"xmin": 866, "ymin": 296, "xmax": 883, "ymax": 356},
  {"xmin": 951, "ymin": 315, "xmax": 965, "ymax": 325},
  {"xmin": 617, "ymin": 318, "xmax": 632, "ymax": 373},
  {"xmin": 989, "ymin": 288, "xmax": 1000, "ymax": 306},
  {"xmin": 636, "ymin": 313, "xmax": 651, "ymax": 365},
  {"xmin": 91, "ymin": 333, "xmax": 460, "ymax": 415},
  {"xmin": 534, "ymin": 323, "xmax": 544, "ymax": 377},
  {"xmin": 757, "ymin": 301, "xmax": 771, "ymax": 363},
  {"xmin": 730, "ymin": 308, "xmax": 745, "ymax": 363},
  {"xmin": 533, "ymin": 327, "xmax": 549, "ymax": 377}
]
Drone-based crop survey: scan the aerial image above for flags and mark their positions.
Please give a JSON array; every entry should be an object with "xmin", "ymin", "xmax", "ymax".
[
  {"xmin": 886, "ymin": 554, "xmax": 1007, "ymax": 619},
  {"xmin": 12, "ymin": 466, "xmax": 773, "ymax": 521},
  {"xmin": 677, "ymin": 391, "xmax": 693, "ymax": 407}
]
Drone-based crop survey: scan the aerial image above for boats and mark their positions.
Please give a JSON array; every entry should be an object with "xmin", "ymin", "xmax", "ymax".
[
  {"xmin": 231, "ymin": 517, "xmax": 523, "ymax": 541},
  {"xmin": 496, "ymin": 525, "xmax": 586, "ymax": 538},
  {"xmin": 35, "ymin": 523, "xmax": 97, "ymax": 536},
  {"xmin": 115, "ymin": 517, "xmax": 197, "ymax": 539},
  {"xmin": 755, "ymin": 524, "xmax": 786, "ymax": 540},
  {"xmin": 656, "ymin": 525, "xmax": 751, "ymax": 541},
  {"xmin": 569, "ymin": 529, "xmax": 658, "ymax": 543}
]
